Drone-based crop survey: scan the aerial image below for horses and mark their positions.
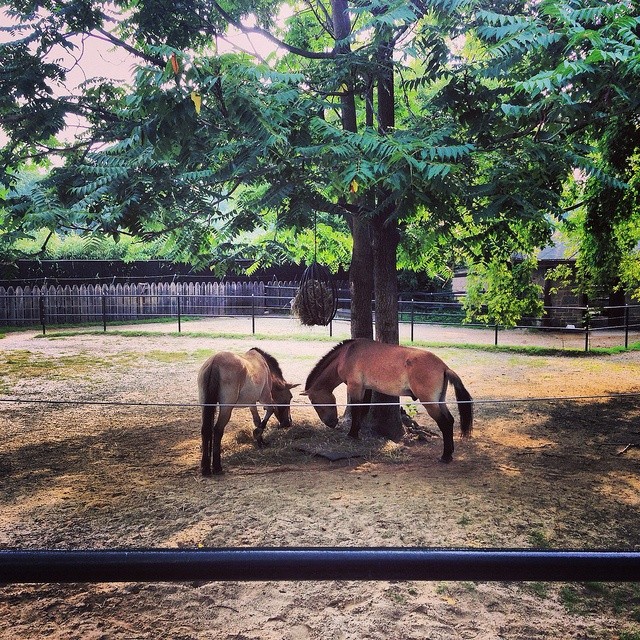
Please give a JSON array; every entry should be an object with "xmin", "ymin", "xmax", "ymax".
[
  {"xmin": 197, "ymin": 346, "xmax": 302, "ymax": 477},
  {"xmin": 298, "ymin": 337, "xmax": 474, "ymax": 464}
]
